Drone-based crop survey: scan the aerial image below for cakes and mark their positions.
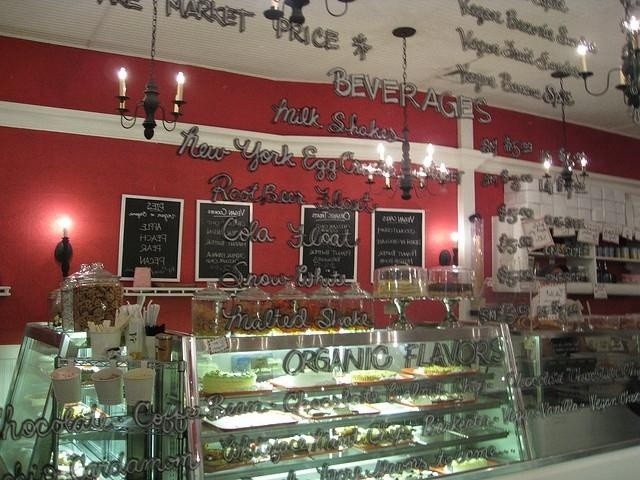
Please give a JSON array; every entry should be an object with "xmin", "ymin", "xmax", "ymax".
[
  {"xmin": 202, "ymin": 369, "xmax": 487, "ymax": 480},
  {"xmin": 428, "ymin": 281, "xmax": 474, "ymax": 298},
  {"xmin": 377, "ymin": 280, "xmax": 423, "ymax": 298}
]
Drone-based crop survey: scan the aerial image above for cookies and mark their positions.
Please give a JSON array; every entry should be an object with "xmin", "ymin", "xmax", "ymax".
[{"xmin": 74, "ymin": 285, "xmax": 122, "ymax": 332}]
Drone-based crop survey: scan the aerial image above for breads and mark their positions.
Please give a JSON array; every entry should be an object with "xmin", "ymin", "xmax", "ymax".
[{"xmin": 516, "ymin": 315, "xmax": 561, "ymax": 330}]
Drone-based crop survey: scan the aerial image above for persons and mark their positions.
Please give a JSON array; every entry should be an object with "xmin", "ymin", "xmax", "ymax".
[{"xmin": 527, "ymin": 263, "xmax": 599, "ymax": 408}]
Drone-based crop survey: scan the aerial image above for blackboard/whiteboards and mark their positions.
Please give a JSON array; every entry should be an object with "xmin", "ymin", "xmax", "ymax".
[
  {"xmin": 116, "ymin": 194, "xmax": 254, "ymax": 282},
  {"xmin": 298, "ymin": 206, "xmax": 426, "ymax": 282}
]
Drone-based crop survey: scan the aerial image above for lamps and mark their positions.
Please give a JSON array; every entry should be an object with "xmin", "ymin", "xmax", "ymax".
[
  {"xmin": 116, "ymin": 0, "xmax": 187, "ymax": 140},
  {"xmin": 533, "ymin": 0, "xmax": 640, "ymax": 200},
  {"xmin": 263, "ymin": 0, "xmax": 351, "ymax": 25},
  {"xmin": 365, "ymin": 26, "xmax": 450, "ymax": 200}
]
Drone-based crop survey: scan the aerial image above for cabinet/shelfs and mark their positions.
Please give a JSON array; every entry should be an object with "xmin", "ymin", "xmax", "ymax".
[
  {"xmin": 1, "ymin": 314, "xmax": 640, "ymax": 480},
  {"xmin": 529, "ymin": 226, "xmax": 639, "ymax": 295}
]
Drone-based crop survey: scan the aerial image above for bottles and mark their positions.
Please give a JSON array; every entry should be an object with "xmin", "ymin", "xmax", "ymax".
[
  {"xmin": 48, "ymin": 262, "xmax": 124, "ymax": 333},
  {"xmin": 154, "ymin": 332, "xmax": 172, "ymax": 363},
  {"xmin": 190, "ymin": 279, "xmax": 375, "ymax": 337}
]
